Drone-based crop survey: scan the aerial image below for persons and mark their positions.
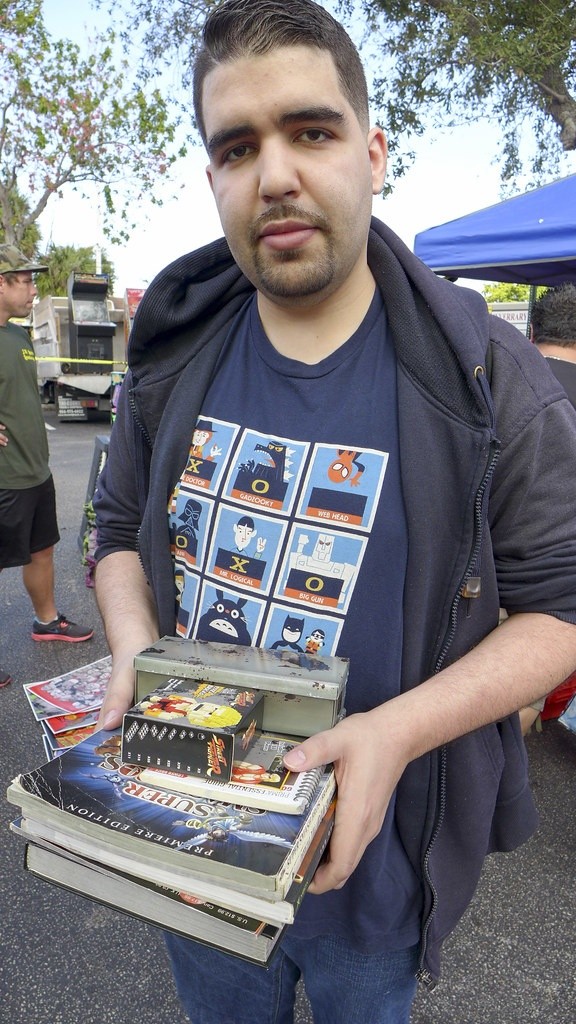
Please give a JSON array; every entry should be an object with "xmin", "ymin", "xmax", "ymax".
[
  {"xmin": 0, "ymin": 242, "xmax": 93, "ymax": 688},
  {"xmin": 519, "ymin": 284, "xmax": 576, "ymax": 740},
  {"xmin": 77, "ymin": 0, "xmax": 576, "ymax": 1024}
]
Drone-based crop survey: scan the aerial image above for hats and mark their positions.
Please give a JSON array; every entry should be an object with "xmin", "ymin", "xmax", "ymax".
[{"xmin": 0, "ymin": 244, "xmax": 49, "ymax": 274}]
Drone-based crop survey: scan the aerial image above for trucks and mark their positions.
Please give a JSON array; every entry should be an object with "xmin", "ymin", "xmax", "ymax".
[{"xmin": 30, "ymin": 271, "xmax": 126, "ymax": 421}]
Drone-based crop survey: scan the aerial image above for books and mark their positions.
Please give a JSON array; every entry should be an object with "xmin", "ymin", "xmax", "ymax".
[
  {"xmin": 7, "ymin": 725, "xmax": 338, "ymax": 968},
  {"xmin": 22, "ymin": 654, "xmax": 113, "ymax": 763}
]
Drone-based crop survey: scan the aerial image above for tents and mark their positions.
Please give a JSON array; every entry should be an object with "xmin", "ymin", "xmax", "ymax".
[{"xmin": 413, "ymin": 172, "xmax": 576, "ymax": 281}]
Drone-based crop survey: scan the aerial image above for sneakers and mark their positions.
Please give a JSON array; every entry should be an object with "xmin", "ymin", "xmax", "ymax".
[
  {"xmin": 31, "ymin": 612, "xmax": 95, "ymax": 642},
  {"xmin": 0, "ymin": 671, "xmax": 12, "ymax": 686}
]
